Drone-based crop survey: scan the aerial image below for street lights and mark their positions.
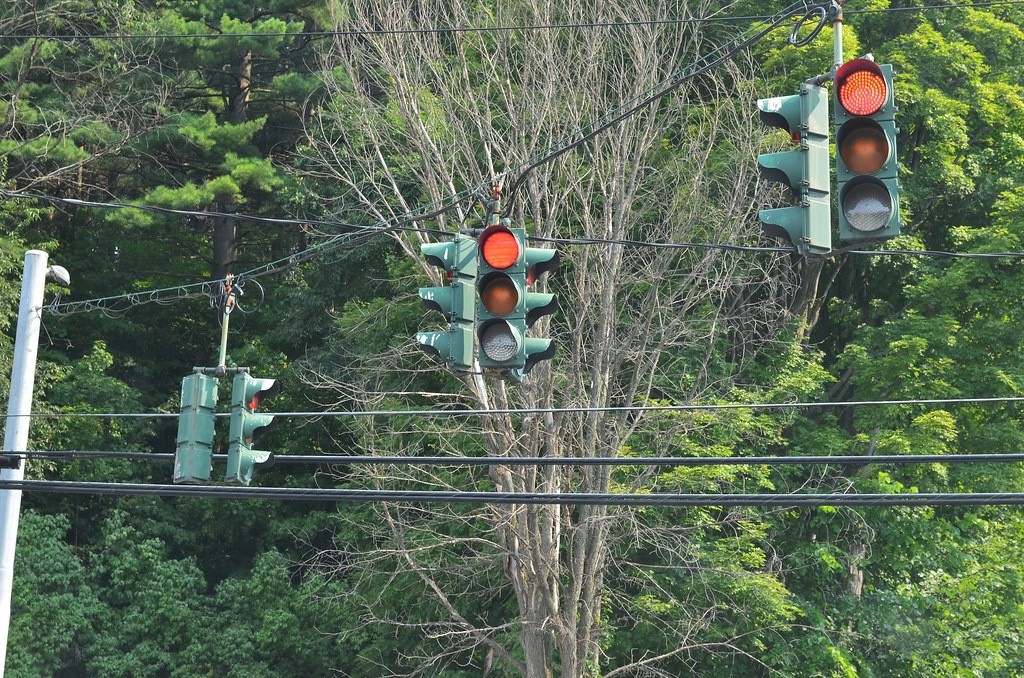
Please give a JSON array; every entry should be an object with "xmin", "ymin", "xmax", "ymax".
[{"xmin": 1, "ymin": 248, "xmax": 71, "ymax": 674}]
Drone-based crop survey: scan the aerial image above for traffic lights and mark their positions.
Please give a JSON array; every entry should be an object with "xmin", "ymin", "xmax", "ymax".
[
  {"xmin": 831, "ymin": 58, "xmax": 903, "ymax": 245},
  {"xmin": 224, "ymin": 371, "xmax": 281, "ymax": 486},
  {"xmin": 510, "ymin": 248, "xmax": 561, "ymax": 383},
  {"xmin": 474, "ymin": 224, "xmax": 525, "ymax": 368},
  {"xmin": 752, "ymin": 81, "xmax": 832, "ymax": 260},
  {"xmin": 416, "ymin": 231, "xmax": 476, "ymax": 373}
]
